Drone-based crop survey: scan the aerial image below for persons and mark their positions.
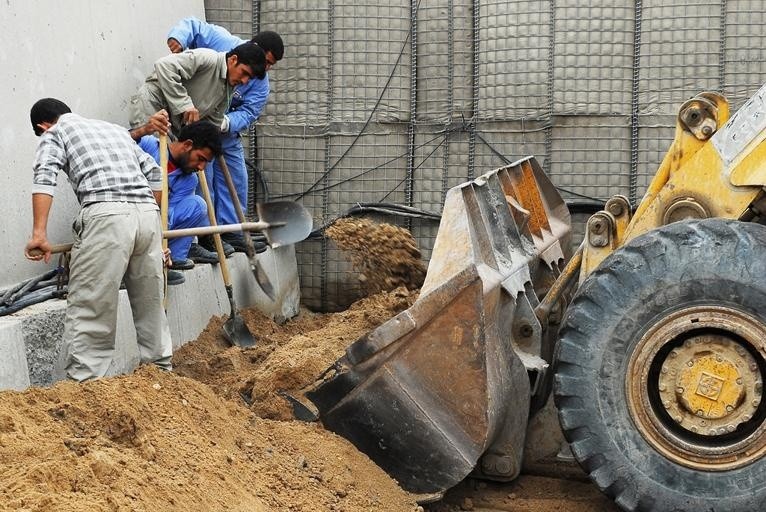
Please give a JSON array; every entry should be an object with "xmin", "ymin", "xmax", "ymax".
[
  {"xmin": 166, "ymin": 15, "xmax": 285, "ymax": 255},
  {"xmin": 126, "ymin": 106, "xmax": 225, "ymax": 286},
  {"xmin": 128, "ymin": 42, "xmax": 266, "ymax": 265},
  {"xmin": 23, "ymin": 96, "xmax": 174, "ymax": 381}
]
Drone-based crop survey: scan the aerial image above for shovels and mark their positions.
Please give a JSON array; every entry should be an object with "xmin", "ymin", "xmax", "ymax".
[
  {"xmin": 28, "ymin": 201, "xmax": 313, "ymax": 257},
  {"xmin": 198, "ymin": 169, "xmax": 254, "ymax": 348},
  {"xmin": 218, "ymin": 155, "xmax": 275, "ymax": 302}
]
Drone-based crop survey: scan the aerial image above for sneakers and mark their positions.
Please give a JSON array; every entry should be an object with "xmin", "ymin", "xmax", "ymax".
[{"xmin": 166, "ymin": 238, "xmax": 267, "ymax": 285}]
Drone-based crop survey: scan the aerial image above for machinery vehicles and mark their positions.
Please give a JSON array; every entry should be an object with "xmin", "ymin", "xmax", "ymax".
[{"xmin": 239, "ymin": 82, "xmax": 765, "ymax": 510}]
